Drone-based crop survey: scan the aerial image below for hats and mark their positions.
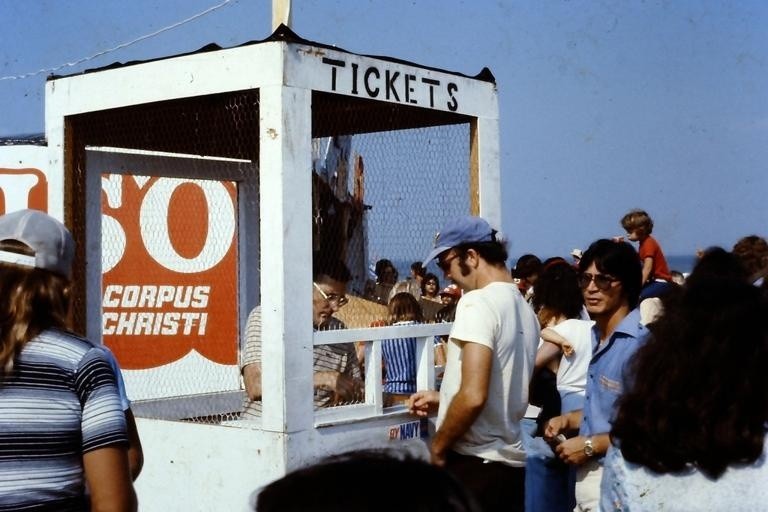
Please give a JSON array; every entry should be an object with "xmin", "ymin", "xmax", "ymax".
[
  {"xmin": 570, "ymin": 249, "xmax": 583, "ymax": 258},
  {"xmin": 438, "ymin": 284, "xmax": 460, "ymax": 297},
  {"xmin": 422, "ymin": 217, "xmax": 493, "ymax": 267},
  {"xmin": 0, "ymin": 210, "xmax": 75, "ymax": 275}
]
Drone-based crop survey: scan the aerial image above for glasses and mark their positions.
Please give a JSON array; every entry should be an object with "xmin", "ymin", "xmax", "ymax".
[
  {"xmin": 437, "ymin": 253, "xmax": 464, "ymax": 271},
  {"xmin": 426, "ymin": 282, "xmax": 436, "ymax": 285},
  {"xmin": 313, "ymin": 283, "xmax": 348, "ymax": 307},
  {"xmin": 579, "ymin": 274, "xmax": 619, "ymax": 289}
]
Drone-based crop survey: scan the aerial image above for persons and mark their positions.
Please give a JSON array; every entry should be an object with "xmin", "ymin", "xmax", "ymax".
[
  {"xmin": 0, "ymin": 208, "xmax": 138, "ymax": 511},
  {"xmin": 77, "ymin": 337, "xmax": 143, "ymax": 482},
  {"xmin": 240, "ymin": 247, "xmax": 386, "ymax": 423},
  {"xmin": 357, "ymin": 209, "xmax": 767, "ymax": 512},
  {"xmin": 249, "ymin": 441, "xmax": 480, "ymax": 512}
]
